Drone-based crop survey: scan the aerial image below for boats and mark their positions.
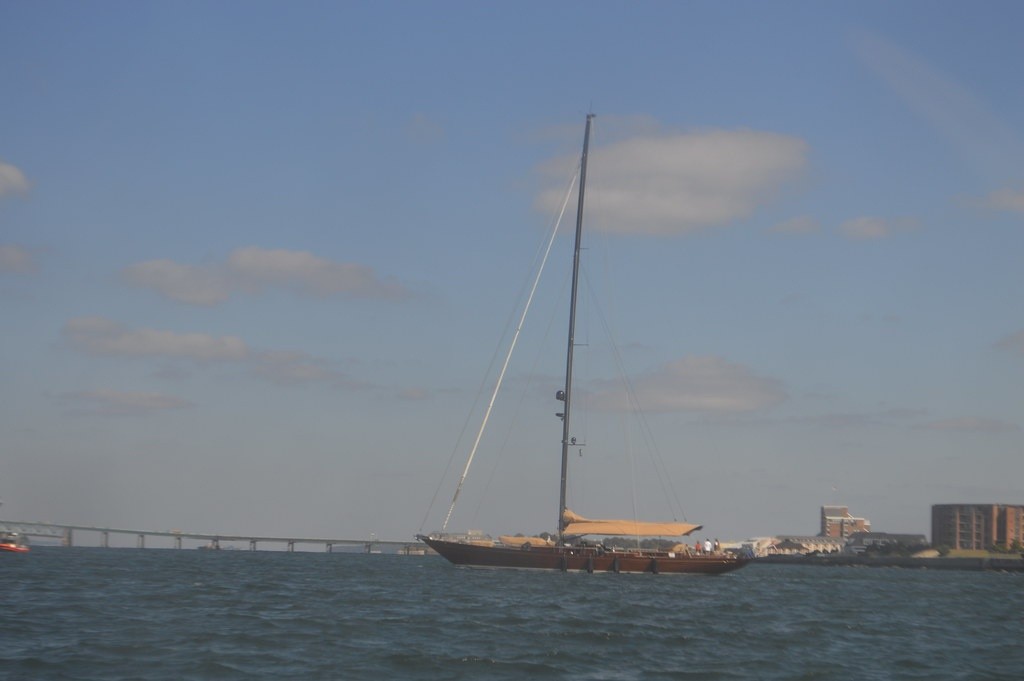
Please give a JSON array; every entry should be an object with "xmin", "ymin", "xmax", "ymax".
[{"xmin": 0, "ymin": 529, "xmax": 31, "ymax": 554}]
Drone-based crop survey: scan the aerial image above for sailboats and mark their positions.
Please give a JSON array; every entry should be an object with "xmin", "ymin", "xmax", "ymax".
[{"xmin": 414, "ymin": 112, "xmax": 750, "ymax": 577}]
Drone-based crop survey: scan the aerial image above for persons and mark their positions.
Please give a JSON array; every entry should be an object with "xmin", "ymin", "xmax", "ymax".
[
  {"xmin": 713, "ymin": 539, "xmax": 720, "ymax": 555},
  {"xmin": 695, "ymin": 540, "xmax": 702, "ymax": 556},
  {"xmin": 596, "ymin": 544, "xmax": 605, "ymax": 557},
  {"xmin": 704, "ymin": 539, "xmax": 712, "ymax": 555}
]
